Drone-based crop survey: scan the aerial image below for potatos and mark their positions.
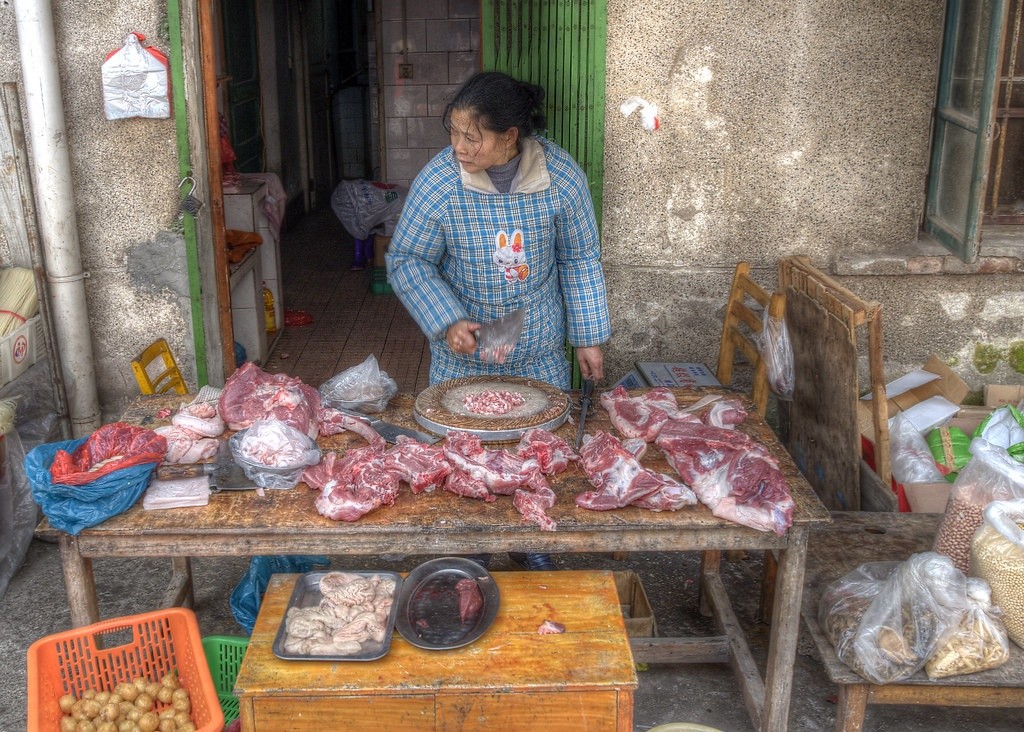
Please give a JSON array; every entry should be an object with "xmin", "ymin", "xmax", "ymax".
[{"xmin": 57, "ymin": 673, "xmax": 196, "ymax": 732}]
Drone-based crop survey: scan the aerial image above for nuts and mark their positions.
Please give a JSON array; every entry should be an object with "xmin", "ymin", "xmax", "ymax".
[{"xmin": 931, "ymin": 495, "xmax": 985, "ymax": 576}]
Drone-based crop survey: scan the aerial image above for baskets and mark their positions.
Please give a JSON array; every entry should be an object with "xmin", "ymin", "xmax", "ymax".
[{"xmin": 28, "ymin": 607, "xmax": 252, "ymax": 732}]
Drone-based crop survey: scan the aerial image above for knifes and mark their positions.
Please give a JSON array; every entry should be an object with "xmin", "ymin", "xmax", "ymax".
[
  {"xmin": 156, "ymin": 462, "xmax": 293, "ymax": 489},
  {"xmin": 471, "ymin": 307, "xmax": 523, "ymax": 346},
  {"xmin": 336, "ymin": 406, "xmax": 443, "ymax": 447}
]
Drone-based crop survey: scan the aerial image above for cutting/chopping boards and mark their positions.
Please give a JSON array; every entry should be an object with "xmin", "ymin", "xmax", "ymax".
[{"xmin": 414, "ymin": 375, "xmax": 572, "ymax": 441}]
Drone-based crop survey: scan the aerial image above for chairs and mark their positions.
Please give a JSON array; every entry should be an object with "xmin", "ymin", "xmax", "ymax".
[
  {"xmin": 716, "ymin": 259, "xmax": 788, "ymax": 420},
  {"xmin": 127, "ymin": 336, "xmax": 191, "ymax": 397}
]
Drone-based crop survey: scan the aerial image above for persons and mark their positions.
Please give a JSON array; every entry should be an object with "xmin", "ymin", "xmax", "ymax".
[{"xmin": 385, "ymin": 70, "xmax": 613, "ymax": 570}]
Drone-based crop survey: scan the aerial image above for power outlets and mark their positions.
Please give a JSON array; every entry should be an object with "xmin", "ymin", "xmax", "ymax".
[{"xmin": 399, "ymin": 64, "xmax": 414, "ymax": 79}]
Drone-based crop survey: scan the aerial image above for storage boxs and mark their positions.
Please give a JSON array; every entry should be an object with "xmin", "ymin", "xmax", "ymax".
[
  {"xmin": 373, "ymin": 236, "xmax": 392, "ymax": 266},
  {"xmin": 857, "ymin": 351, "xmax": 1024, "ymax": 510},
  {"xmin": 612, "ymin": 568, "xmax": 659, "ymax": 674},
  {"xmin": 0, "ymin": 314, "xmax": 48, "ymax": 391}
]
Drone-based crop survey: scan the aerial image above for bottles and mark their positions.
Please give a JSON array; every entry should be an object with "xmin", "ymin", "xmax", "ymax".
[{"xmin": 262, "ymin": 281, "xmax": 276, "ymax": 337}]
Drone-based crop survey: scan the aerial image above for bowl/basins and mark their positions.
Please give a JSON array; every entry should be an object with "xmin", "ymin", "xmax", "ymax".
[{"xmin": 231, "ymin": 429, "xmax": 316, "ymax": 476}]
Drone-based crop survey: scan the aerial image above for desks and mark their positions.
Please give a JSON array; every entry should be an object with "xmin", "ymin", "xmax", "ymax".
[
  {"xmin": 764, "ymin": 507, "xmax": 1024, "ymax": 732},
  {"xmin": 229, "ymin": 565, "xmax": 641, "ymax": 732},
  {"xmin": 37, "ymin": 388, "xmax": 835, "ymax": 732}
]
